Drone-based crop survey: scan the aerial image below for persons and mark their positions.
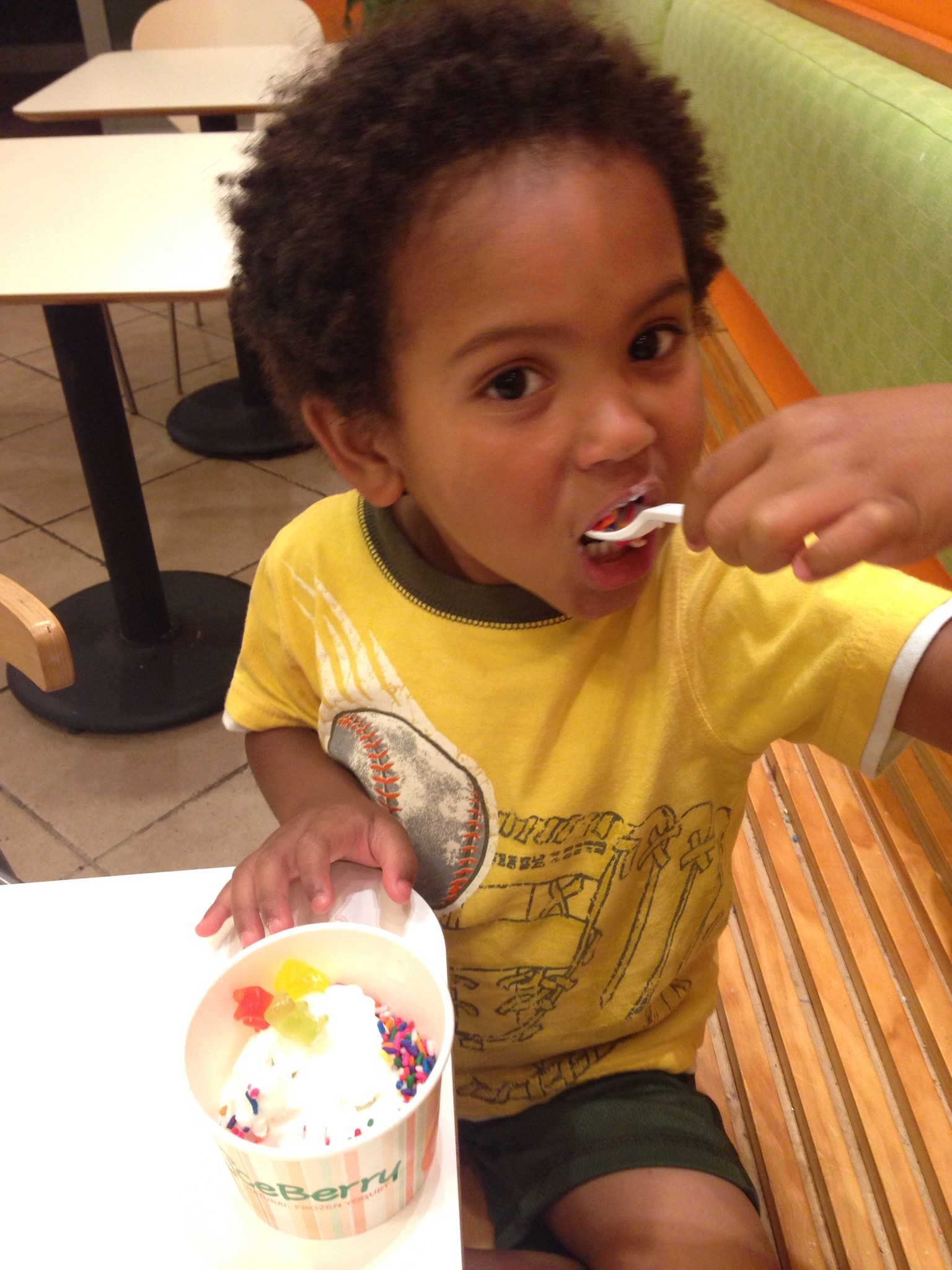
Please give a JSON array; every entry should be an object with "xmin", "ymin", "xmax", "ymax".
[{"xmin": 194, "ymin": 1, "xmax": 952, "ymax": 1270}]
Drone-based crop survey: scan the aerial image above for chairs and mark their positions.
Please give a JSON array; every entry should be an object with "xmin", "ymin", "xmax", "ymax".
[{"xmin": 128, "ymin": 0, "xmax": 327, "ymax": 51}]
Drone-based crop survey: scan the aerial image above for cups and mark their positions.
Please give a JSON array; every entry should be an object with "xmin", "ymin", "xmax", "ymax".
[{"xmin": 184, "ymin": 923, "xmax": 447, "ymax": 1238}]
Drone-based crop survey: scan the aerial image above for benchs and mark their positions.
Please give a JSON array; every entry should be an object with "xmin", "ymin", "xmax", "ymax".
[{"xmin": 456, "ymin": 0, "xmax": 952, "ymax": 1270}]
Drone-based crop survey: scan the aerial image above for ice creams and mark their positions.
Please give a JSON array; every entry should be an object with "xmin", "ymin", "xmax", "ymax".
[{"xmin": 220, "ymin": 959, "xmax": 437, "ymax": 1148}]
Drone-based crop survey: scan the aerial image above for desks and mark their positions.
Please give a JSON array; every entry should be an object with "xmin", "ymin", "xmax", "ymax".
[
  {"xmin": 0, "ymin": 131, "xmax": 265, "ymax": 740},
  {"xmin": 11, "ymin": 46, "xmax": 337, "ymax": 465},
  {"xmin": 0, "ymin": 860, "xmax": 468, "ymax": 1270}
]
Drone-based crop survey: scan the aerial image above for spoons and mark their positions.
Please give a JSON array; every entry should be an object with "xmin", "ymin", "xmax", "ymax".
[{"xmin": 585, "ymin": 504, "xmax": 684, "ymax": 541}]
